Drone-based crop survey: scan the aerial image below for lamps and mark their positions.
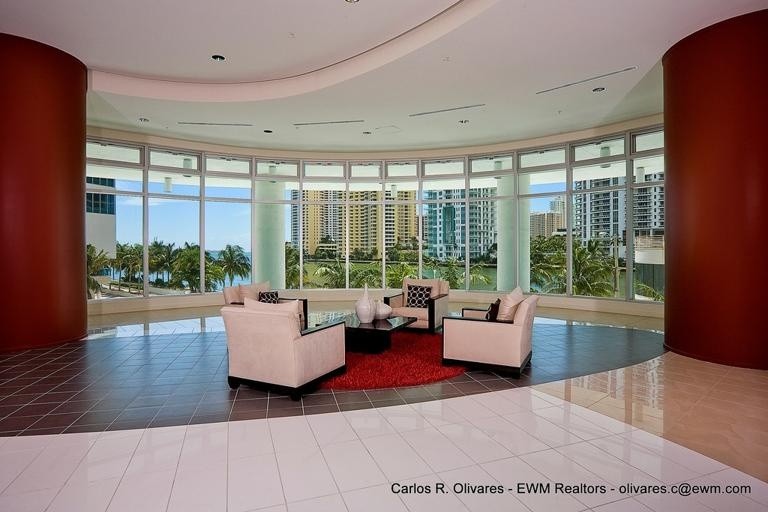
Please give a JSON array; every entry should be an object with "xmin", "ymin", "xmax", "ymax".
[
  {"xmin": 164, "ymin": 177, "xmax": 172, "ymax": 193},
  {"xmin": 636, "ymin": 167, "xmax": 645, "ymax": 184},
  {"xmin": 494, "ymin": 160, "xmax": 502, "ymax": 179},
  {"xmin": 268, "ymin": 165, "xmax": 277, "ymax": 183},
  {"xmin": 599, "ymin": 141, "xmax": 611, "ymax": 168},
  {"xmin": 391, "ymin": 183, "xmax": 397, "ymax": 198},
  {"xmin": 182, "ymin": 157, "xmax": 192, "ymax": 177}
]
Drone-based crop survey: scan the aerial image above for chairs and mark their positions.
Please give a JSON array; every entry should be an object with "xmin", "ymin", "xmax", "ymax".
[
  {"xmin": 383, "ymin": 281, "xmax": 450, "ymax": 336},
  {"xmin": 222, "ymin": 286, "xmax": 308, "ymax": 331},
  {"xmin": 218, "ymin": 305, "xmax": 348, "ymax": 402},
  {"xmin": 441, "ymin": 295, "xmax": 540, "ymax": 380}
]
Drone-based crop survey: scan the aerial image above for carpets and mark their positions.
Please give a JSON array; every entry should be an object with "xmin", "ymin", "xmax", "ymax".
[{"xmin": 319, "ymin": 332, "xmax": 466, "ymax": 391}]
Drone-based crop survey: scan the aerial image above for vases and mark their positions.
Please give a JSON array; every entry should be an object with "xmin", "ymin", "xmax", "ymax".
[
  {"xmin": 374, "ymin": 296, "xmax": 392, "ymax": 319},
  {"xmin": 355, "ymin": 283, "xmax": 376, "ymax": 323}
]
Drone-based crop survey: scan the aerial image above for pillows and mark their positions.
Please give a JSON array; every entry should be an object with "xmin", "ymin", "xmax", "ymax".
[
  {"xmin": 239, "ymin": 280, "xmax": 302, "ymax": 334},
  {"xmin": 402, "ymin": 276, "xmax": 440, "ymax": 307},
  {"xmin": 496, "ymin": 286, "xmax": 524, "ymax": 323},
  {"xmin": 406, "ymin": 283, "xmax": 434, "ymax": 309},
  {"xmin": 484, "ymin": 297, "xmax": 501, "ymax": 321}
]
